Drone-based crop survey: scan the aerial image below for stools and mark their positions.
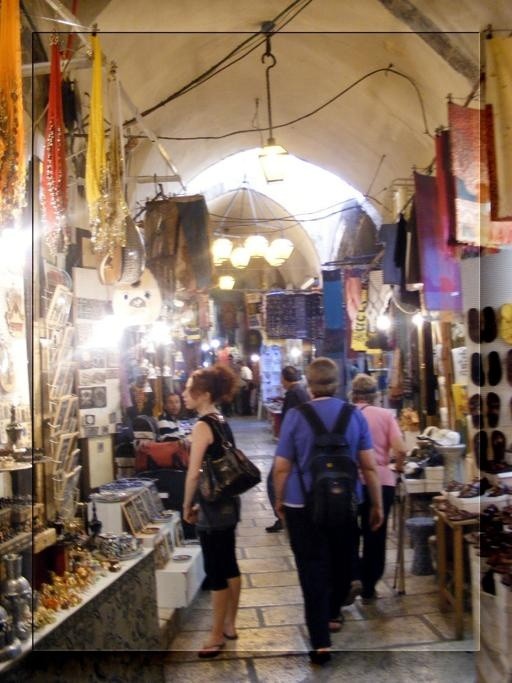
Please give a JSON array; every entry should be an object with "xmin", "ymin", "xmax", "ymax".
[{"xmin": 406, "ymin": 518, "xmax": 435, "ymax": 576}]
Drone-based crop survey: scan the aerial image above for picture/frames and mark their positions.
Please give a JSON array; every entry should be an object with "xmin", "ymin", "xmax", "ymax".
[{"xmin": 37, "ymin": 284, "xmax": 165, "ymax": 536}]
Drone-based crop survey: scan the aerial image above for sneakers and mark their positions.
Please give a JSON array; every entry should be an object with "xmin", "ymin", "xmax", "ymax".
[
  {"xmin": 436, "ymin": 476, "xmax": 512, "ymax": 586},
  {"xmin": 264, "ymin": 518, "xmax": 282, "ymax": 533},
  {"xmin": 305, "ymin": 578, "xmax": 384, "ymax": 664}
]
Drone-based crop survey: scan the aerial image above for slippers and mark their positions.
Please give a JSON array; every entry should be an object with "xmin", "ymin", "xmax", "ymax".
[
  {"xmin": 223, "ymin": 630, "xmax": 240, "ymax": 640},
  {"xmin": 199, "ymin": 639, "xmax": 226, "ymax": 658},
  {"xmin": 466, "ymin": 300, "xmax": 512, "ymax": 473}
]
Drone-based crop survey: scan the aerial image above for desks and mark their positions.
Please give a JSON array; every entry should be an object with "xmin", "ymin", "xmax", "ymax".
[
  {"xmin": 393, "ymin": 482, "xmax": 442, "ymax": 594},
  {"xmin": 469, "ymin": 545, "xmax": 512, "ymax": 683},
  {"xmin": 431, "ymin": 504, "xmax": 479, "ymax": 640},
  {"xmin": 0, "ymin": 548, "xmax": 165, "ymax": 683}
]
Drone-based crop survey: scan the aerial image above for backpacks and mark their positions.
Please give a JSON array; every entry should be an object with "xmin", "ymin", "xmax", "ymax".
[{"xmin": 288, "ymin": 402, "xmax": 362, "ymax": 532}]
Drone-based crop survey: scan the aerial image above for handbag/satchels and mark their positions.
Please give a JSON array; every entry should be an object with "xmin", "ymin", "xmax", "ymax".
[
  {"xmin": 110, "ymin": 265, "xmax": 163, "ymax": 327},
  {"xmin": 94, "ymin": 210, "xmax": 148, "ymax": 286},
  {"xmin": 190, "ymin": 415, "xmax": 265, "ymax": 505}
]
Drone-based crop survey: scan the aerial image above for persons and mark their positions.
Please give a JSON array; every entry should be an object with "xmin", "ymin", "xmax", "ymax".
[
  {"xmin": 264, "ymin": 364, "xmax": 311, "ymax": 531},
  {"xmin": 158, "ymin": 392, "xmax": 182, "ymax": 442},
  {"xmin": 129, "ymin": 363, "xmax": 156, "ymax": 423},
  {"xmin": 271, "ymin": 356, "xmax": 387, "ymax": 665},
  {"xmin": 182, "ymin": 364, "xmax": 242, "ymax": 657},
  {"xmin": 237, "ymin": 360, "xmax": 255, "ymax": 416},
  {"xmin": 224, "ymin": 354, "xmax": 241, "ymax": 417},
  {"xmin": 343, "ymin": 373, "xmax": 409, "ymax": 605}
]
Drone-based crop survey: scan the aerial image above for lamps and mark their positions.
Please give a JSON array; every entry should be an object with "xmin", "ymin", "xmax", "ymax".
[
  {"xmin": 260, "ymin": 53, "xmax": 288, "ymax": 182},
  {"xmin": 211, "ymin": 177, "xmax": 294, "ymax": 267},
  {"xmin": 218, "ymin": 263, "xmax": 234, "ymax": 290}
]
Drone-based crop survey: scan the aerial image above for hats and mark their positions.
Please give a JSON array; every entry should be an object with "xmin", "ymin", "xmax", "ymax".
[
  {"xmin": 413, "ymin": 427, "xmax": 461, "ymax": 447},
  {"xmin": 305, "ymin": 357, "xmax": 340, "ymax": 395},
  {"xmin": 345, "ymin": 373, "xmax": 387, "ymax": 398},
  {"xmin": 154, "ymin": 419, "xmax": 193, "ymax": 443}
]
